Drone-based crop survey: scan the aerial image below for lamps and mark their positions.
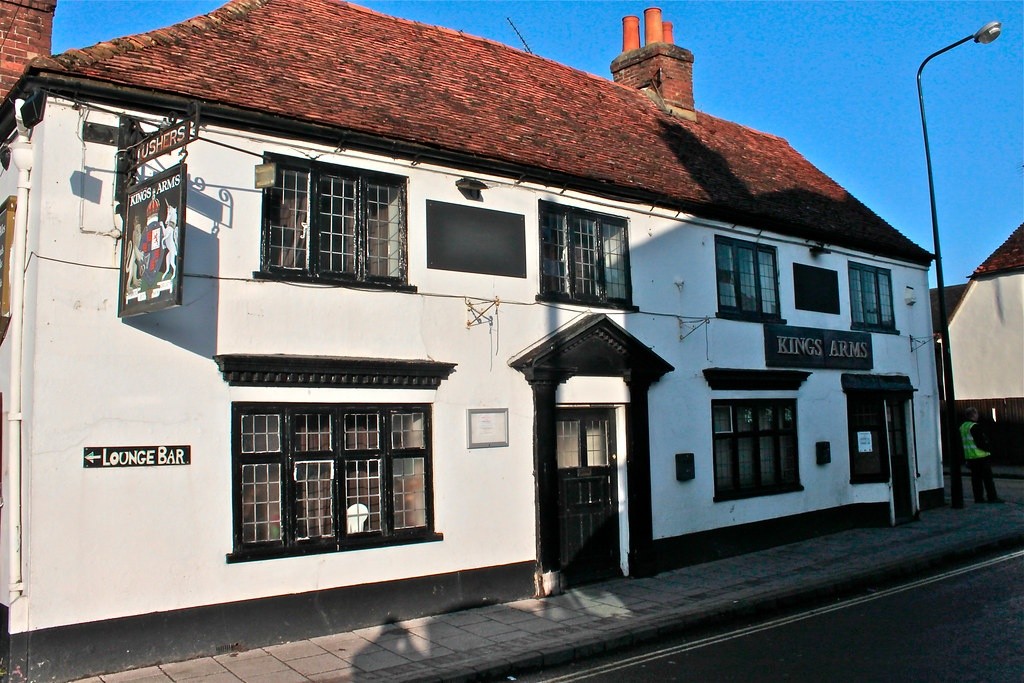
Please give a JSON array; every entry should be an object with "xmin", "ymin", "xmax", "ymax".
[
  {"xmin": 810, "ymin": 247, "xmax": 831, "ymax": 258},
  {"xmin": 456, "ymin": 179, "xmax": 487, "ymax": 199}
]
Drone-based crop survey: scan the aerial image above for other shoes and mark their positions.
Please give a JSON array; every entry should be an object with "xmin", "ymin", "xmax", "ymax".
[
  {"xmin": 975, "ymin": 499, "xmax": 987, "ymax": 503},
  {"xmin": 994, "ymin": 498, "xmax": 1005, "ymax": 503}
]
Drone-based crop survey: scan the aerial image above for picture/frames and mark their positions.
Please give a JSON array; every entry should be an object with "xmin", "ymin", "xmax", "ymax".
[{"xmin": 466, "ymin": 408, "xmax": 510, "ymax": 449}]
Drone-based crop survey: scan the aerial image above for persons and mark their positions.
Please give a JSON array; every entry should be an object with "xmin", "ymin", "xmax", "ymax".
[{"xmin": 960, "ymin": 407, "xmax": 1005, "ymax": 504}]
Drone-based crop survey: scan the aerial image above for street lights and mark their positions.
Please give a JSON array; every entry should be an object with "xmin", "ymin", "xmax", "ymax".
[{"xmin": 916, "ymin": 22, "xmax": 1001, "ymax": 509}]
